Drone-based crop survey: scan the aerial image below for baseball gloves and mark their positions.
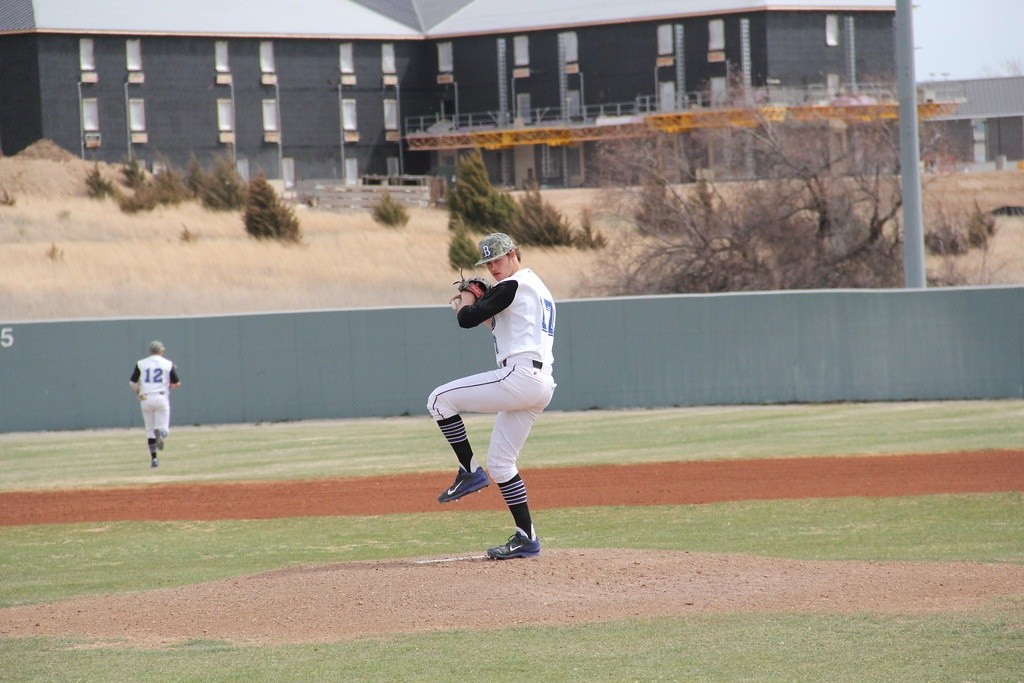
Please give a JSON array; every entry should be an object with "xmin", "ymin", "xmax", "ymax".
[{"xmin": 457, "ymin": 276, "xmax": 492, "ymax": 303}]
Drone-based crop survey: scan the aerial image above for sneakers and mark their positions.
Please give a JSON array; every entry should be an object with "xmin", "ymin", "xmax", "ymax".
[
  {"xmin": 438, "ymin": 467, "xmax": 490, "ymax": 503},
  {"xmin": 487, "ymin": 531, "xmax": 540, "ymax": 559}
]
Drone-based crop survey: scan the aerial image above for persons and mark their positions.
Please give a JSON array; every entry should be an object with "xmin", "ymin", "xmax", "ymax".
[
  {"xmin": 130, "ymin": 342, "xmax": 180, "ymax": 467},
  {"xmin": 427, "ymin": 233, "xmax": 556, "ymax": 560}
]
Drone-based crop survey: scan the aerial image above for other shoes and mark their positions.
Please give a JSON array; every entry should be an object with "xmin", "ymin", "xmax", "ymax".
[
  {"xmin": 154, "ymin": 429, "xmax": 164, "ymax": 450},
  {"xmin": 151, "ymin": 459, "xmax": 159, "ymax": 467}
]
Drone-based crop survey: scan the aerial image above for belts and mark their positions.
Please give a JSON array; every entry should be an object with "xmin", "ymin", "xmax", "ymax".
[
  {"xmin": 144, "ymin": 392, "xmax": 164, "ymax": 396},
  {"xmin": 503, "ymin": 358, "xmax": 544, "ymax": 370}
]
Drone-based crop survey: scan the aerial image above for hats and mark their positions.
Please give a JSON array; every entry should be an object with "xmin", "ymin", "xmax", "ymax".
[
  {"xmin": 149, "ymin": 341, "xmax": 165, "ymax": 351},
  {"xmin": 475, "ymin": 233, "xmax": 515, "ymax": 266}
]
